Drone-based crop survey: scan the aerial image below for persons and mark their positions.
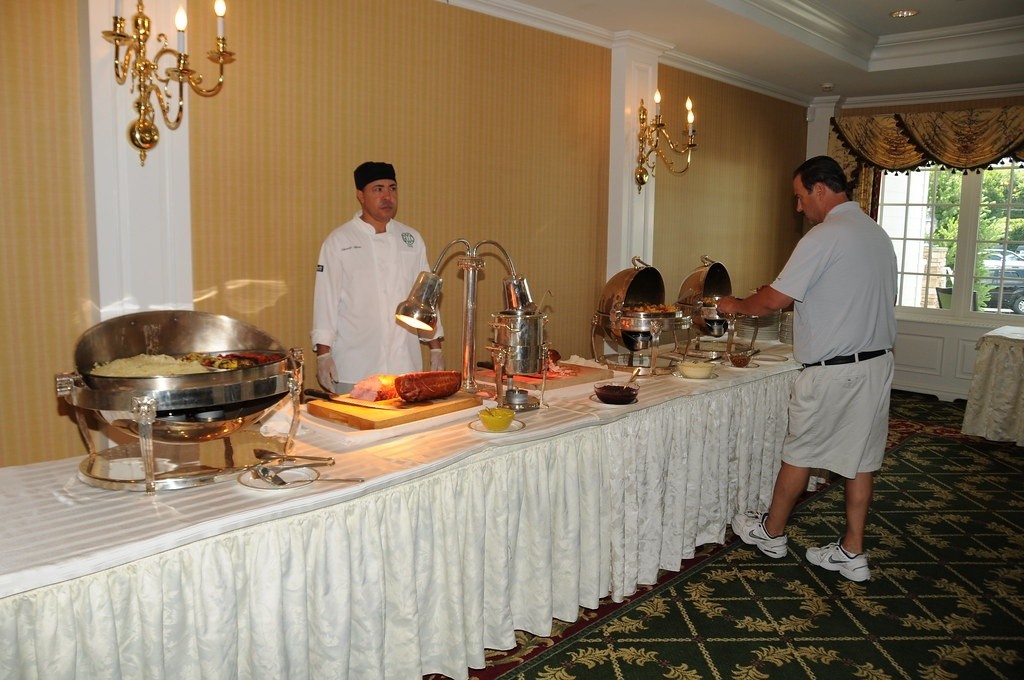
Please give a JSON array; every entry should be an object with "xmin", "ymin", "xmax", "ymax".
[
  {"xmin": 312, "ymin": 161, "xmax": 447, "ymax": 397},
  {"xmin": 714, "ymin": 155, "xmax": 899, "ymax": 583}
]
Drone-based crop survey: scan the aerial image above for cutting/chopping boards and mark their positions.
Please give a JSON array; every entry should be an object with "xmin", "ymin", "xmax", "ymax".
[
  {"xmin": 474, "ymin": 363, "xmax": 614, "ymax": 391},
  {"xmin": 307, "ymin": 394, "xmax": 483, "ymax": 431}
]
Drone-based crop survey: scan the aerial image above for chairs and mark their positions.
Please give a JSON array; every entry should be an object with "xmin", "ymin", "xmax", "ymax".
[{"xmin": 937, "ymin": 287, "xmax": 979, "ymax": 311}]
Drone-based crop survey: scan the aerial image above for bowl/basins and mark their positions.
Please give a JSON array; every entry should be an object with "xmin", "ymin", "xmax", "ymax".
[
  {"xmin": 478, "ymin": 408, "xmax": 516, "ymax": 431},
  {"xmin": 675, "ymin": 362, "xmax": 716, "ymax": 379},
  {"xmin": 729, "ymin": 355, "xmax": 751, "ymax": 368},
  {"xmin": 594, "ymin": 381, "xmax": 640, "ymax": 405}
]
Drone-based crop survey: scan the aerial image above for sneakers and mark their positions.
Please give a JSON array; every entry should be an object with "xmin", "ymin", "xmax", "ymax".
[
  {"xmin": 731, "ymin": 511, "xmax": 788, "ymax": 559},
  {"xmin": 805, "ymin": 536, "xmax": 871, "ymax": 582}
]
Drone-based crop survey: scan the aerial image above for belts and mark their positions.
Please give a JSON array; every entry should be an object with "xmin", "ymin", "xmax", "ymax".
[{"xmin": 802, "ymin": 349, "xmax": 886, "ymax": 369}]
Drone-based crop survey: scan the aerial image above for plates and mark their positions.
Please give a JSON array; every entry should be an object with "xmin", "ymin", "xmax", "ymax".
[
  {"xmin": 589, "ymin": 394, "xmax": 638, "ymax": 406},
  {"xmin": 751, "ymin": 354, "xmax": 788, "ymax": 364},
  {"xmin": 671, "ymin": 371, "xmax": 719, "ymax": 382},
  {"xmin": 736, "ymin": 310, "xmax": 793, "ymax": 345},
  {"xmin": 720, "ymin": 361, "xmax": 760, "ymax": 370},
  {"xmin": 237, "ymin": 465, "xmax": 320, "ymax": 492},
  {"xmin": 468, "ymin": 419, "xmax": 526, "ymax": 433}
]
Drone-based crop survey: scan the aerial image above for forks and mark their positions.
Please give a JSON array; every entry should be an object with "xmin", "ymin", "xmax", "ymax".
[{"xmin": 255, "ymin": 466, "xmax": 364, "ymax": 487}]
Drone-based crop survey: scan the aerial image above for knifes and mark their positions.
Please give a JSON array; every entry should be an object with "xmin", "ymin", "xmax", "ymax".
[
  {"xmin": 304, "ymin": 389, "xmax": 396, "ymax": 410},
  {"xmin": 477, "ymin": 362, "xmax": 555, "ymax": 379}
]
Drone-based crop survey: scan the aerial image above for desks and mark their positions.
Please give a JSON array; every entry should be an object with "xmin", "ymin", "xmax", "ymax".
[
  {"xmin": 0, "ymin": 335, "xmax": 824, "ymax": 680},
  {"xmin": 960, "ymin": 325, "xmax": 1024, "ymax": 447}
]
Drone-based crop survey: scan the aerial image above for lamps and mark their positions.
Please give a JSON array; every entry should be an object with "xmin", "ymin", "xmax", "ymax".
[
  {"xmin": 396, "ymin": 235, "xmax": 539, "ymax": 397},
  {"xmin": 102, "ymin": 0, "xmax": 234, "ymax": 166},
  {"xmin": 635, "ymin": 88, "xmax": 697, "ymax": 193}
]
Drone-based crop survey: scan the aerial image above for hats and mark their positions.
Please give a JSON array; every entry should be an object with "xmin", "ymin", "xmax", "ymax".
[{"xmin": 354, "ymin": 161, "xmax": 396, "ymax": 190}]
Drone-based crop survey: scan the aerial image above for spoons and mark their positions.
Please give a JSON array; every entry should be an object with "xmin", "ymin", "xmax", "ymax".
[
  {"xmin": 254, "ymin": 449, "xmax": 334, "ymax": 461},
  {"xmin": 252, "ymin": 462, "xmax": 336, "ymax": 479}
]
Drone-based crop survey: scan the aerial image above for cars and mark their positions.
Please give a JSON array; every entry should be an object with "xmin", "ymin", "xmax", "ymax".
[{"xmin": 978, "ymin": 246, "xmax": 1024, "ymax": 315}]
